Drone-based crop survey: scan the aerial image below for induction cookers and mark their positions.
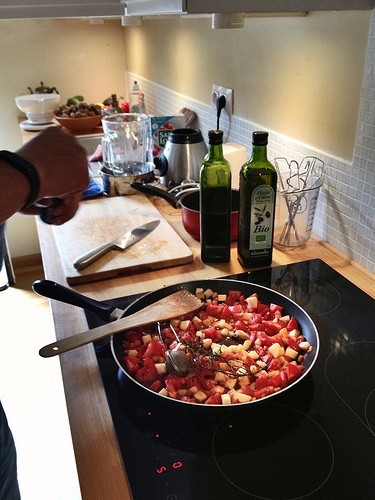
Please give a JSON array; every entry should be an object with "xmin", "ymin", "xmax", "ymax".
[{"xmin": 83, "ymin": 258, "xmax": 374, "ymax": 500}]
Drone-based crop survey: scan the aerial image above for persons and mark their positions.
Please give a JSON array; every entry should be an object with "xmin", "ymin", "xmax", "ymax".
[{"xmin": 0, "ymin": 125, "xmax": 89, "ymax": 500}]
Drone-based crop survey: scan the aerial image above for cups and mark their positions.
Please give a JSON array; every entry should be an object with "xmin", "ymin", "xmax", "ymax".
[
  {"xmin": 274, "ymin": 172, "xmax": 324, "ymax": 247},
  {"xmin": 101, "ymin": 113, "xmax": 152, "ymax": 176}
]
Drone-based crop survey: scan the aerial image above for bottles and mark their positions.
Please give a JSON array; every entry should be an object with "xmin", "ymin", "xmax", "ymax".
[
  {"xmin": 199, "ymin": 130, "xmax": 232, "ymax": 264},
  {"xmin": 238, "ymin": 130, "xmax": 278, "ymax": 269},
  {"xmin": 128, "ymin": 81, "xmax": 146, "ymax": 114}
]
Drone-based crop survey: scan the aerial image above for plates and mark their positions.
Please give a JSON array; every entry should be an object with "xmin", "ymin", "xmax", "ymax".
[{"xmin": 19, "ymin": 120, "xmax": 60, "ymax": 130}]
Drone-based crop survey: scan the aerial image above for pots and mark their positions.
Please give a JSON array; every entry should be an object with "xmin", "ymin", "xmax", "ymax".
[
  {"xmin": 130, "ymin": 181, "xmax": 240, "ymax": 242},
  {"xmin": 31, "ymin": 279, "xmax": 320, "ymax": 410}
]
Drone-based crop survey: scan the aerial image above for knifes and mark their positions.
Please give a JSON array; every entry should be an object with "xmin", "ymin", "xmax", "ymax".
[{"xmin": 73, "ymin": 220, "xmax": 160, "ymax": 270}]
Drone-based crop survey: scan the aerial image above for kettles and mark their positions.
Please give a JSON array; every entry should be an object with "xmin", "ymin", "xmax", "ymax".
[{"xmin": 153, "ymin": 128, "xmax": 208, "ymax": 187}]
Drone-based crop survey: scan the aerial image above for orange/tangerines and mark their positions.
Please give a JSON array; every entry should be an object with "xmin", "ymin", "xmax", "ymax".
[{"xmin": 66, "ymin": 98, "xmax": 78, "ymax": 106}]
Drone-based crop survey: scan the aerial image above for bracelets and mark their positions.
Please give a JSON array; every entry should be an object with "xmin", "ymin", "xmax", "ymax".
[{"xmin": 0, "ymin": 150, "xmax": 40, "ymax": 210}]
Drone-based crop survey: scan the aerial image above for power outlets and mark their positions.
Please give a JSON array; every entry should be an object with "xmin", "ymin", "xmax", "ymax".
[{"xmin": 211, "ymin": 83, "xmax": 233, "ymax": 116}]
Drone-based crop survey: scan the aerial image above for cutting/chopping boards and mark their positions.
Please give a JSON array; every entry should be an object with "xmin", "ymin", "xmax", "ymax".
[{"xmin": 52, "ymin": 194, "xmax": 193, "ymax": 285}]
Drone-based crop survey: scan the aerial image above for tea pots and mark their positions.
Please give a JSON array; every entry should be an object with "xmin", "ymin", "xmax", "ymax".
[{"xmin": 27, "ymin": 81, "xmax": 60, "ymax": 94}]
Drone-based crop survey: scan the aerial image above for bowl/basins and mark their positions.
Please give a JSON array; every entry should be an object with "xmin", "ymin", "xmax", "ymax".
[
  {"xmin": 15, "ymin": 94, "xmax": 60, "ymax": 124},
  {"xmin": 52, "ymin": 110, "xmax": 102, "ymax": 133}
]
